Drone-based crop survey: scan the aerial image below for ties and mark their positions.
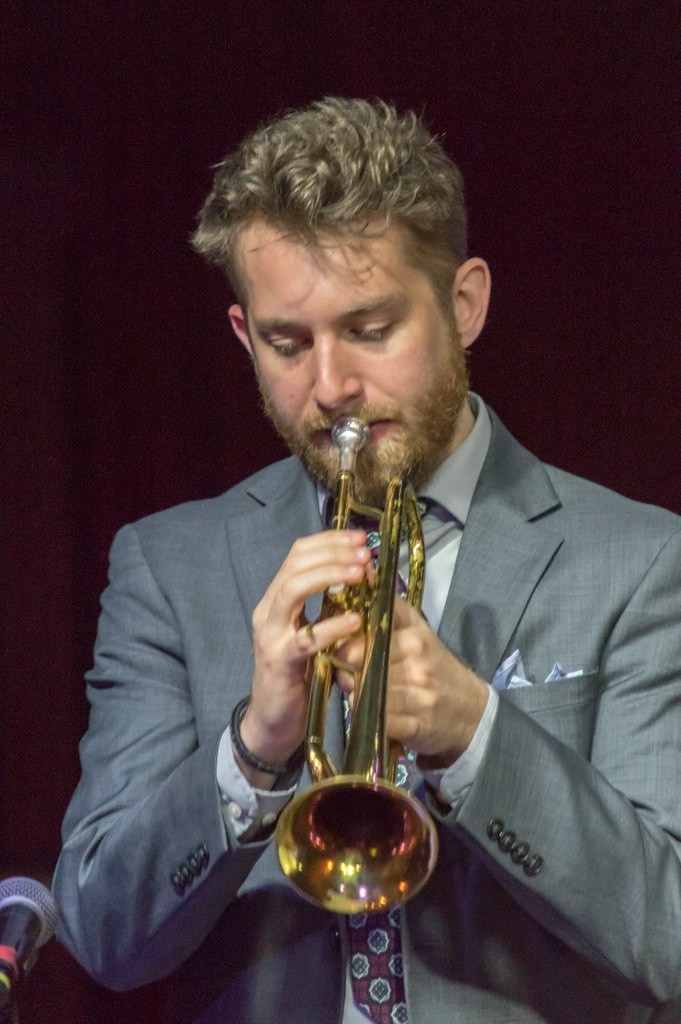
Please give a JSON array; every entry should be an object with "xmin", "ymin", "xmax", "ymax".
[{"xmin": 325, "ymin": 498, "xmax": 431, "ymax": 1024}]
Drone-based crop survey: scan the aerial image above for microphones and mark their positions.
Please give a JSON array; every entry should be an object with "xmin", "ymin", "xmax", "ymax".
[{"xmin": 0, "ymin": 875, "xmax": 57, "ymax": 1010}]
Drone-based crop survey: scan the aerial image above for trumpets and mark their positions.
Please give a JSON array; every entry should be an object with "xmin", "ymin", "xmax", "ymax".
[{"xmin": 274, "ymin": 413, "xmax": 442, "ymax": 916}]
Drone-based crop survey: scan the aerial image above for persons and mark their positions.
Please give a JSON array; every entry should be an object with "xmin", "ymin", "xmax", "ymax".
[{"xmin": 49, "ymin": 97, "xmax": 681, "ymax": 1024}]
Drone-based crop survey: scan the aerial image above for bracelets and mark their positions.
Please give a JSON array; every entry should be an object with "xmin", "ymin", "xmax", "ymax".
[{"xmin": 230, "ymin": 696, "xmax": 289, "ymax": 776}]
